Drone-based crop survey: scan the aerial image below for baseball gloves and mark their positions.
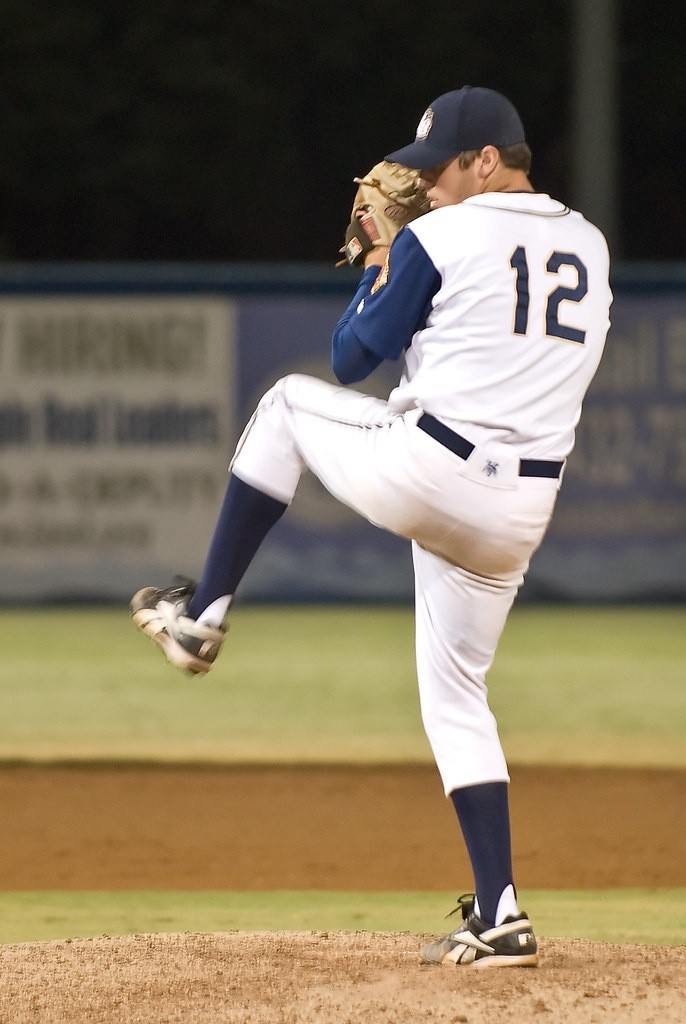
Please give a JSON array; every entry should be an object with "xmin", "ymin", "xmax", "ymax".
[{"xmin": 350, "ymin": 159, "xmax": 432, "ymax": 247}]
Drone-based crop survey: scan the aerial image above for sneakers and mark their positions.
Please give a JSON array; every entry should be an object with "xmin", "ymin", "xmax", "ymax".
[
  {"xmin": 416, "ymin": 885, "xmax": 540, "ymax": 970},
  {"xmin": 130, "ymin": 574, "xmax": 227, "ymax": 675}
]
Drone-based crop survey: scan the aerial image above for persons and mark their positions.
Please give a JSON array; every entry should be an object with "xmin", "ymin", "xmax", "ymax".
[{"xmin": 130, "ymin": 86, "xmax": 613, "ymax": 969}]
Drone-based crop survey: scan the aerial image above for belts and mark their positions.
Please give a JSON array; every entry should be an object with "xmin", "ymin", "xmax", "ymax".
[{"xmin": 416, "ymin": 412, "xmax": 565, "ymax": 481}]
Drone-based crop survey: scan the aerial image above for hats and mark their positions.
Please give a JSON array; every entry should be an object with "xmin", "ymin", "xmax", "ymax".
[{"xmin": 385, "ymin": 86, "xmax": 527, "ymax": 170}]
